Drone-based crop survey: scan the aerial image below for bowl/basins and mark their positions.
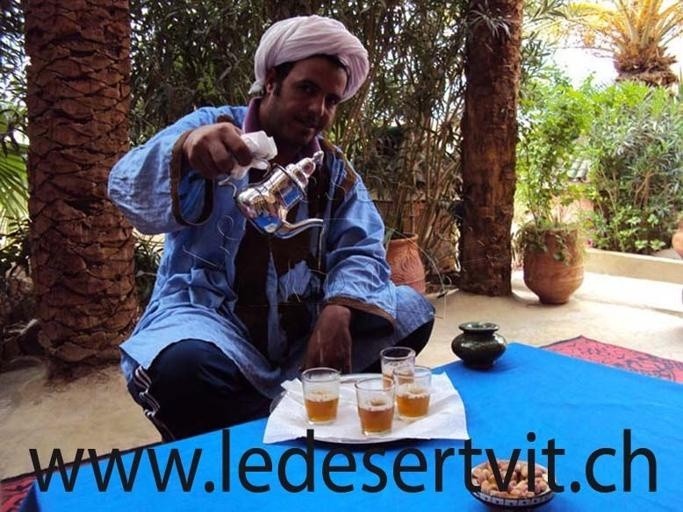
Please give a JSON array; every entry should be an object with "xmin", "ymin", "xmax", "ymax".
[{"xmin": 470, "ymin": 460, "xmax": 556, "ymax": 512}]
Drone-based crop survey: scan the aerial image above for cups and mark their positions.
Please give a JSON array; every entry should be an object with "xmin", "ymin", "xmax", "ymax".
[
  {"xmin": 357, "ymin": 348, "xmax": 431, "ymax": 437},
  {"xmin": 300, "ymin": 366, "xmax": 341, "ymax": 423}
]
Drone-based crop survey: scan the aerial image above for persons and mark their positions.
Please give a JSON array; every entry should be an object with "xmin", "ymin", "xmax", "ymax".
[{"xmin": 105, "ymin": 10, "xmax": 437, "ymax": 443}]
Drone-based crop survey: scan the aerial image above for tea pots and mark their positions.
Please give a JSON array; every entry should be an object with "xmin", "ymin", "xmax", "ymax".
[{"xmin": 220, "ymin": 151, "xmax": 323, "ymax": 239}]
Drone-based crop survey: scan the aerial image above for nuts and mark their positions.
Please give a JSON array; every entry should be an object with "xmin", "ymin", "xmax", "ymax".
[{"xmin": 473, "ymin": 461, "xmax": 547, "ymax": 498}]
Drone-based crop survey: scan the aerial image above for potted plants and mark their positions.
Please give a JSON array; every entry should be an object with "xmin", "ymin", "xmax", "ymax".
[{"xmin": 509, "ymin": 66, "xmax": 623, "ymax": 306}]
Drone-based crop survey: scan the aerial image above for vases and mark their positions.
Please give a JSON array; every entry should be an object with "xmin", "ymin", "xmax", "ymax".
[
  {"xmin": 671, "ymin": 219, "xmax": 683, "ymax": 260},
  {"xmin": 383, "ymin": 233, "xmax": 426, "ymax": 299}
]
